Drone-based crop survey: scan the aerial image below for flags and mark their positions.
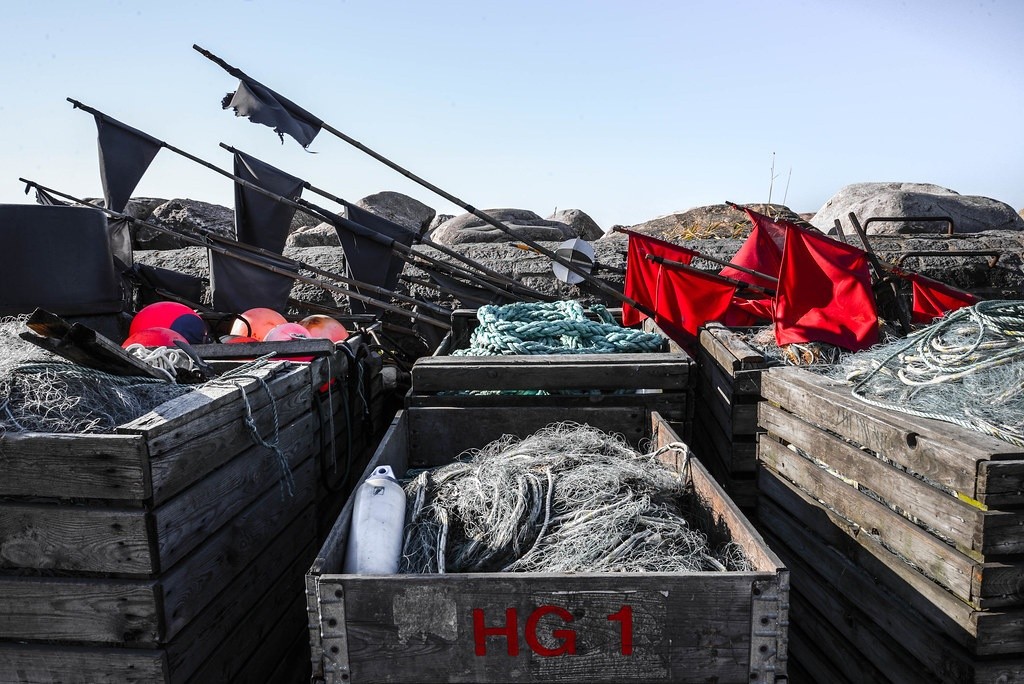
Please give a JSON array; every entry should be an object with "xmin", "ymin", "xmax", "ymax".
[
  {"xmin": 222, "ymin": 79, "xmax": 320, "ymax": 145},
  {"xmin": 0, "ymin": 204, "xmax": 122, "ymax": 321},
  {"xmin": 655, "ymin": 265, "xmax": 734, "ymax": 337},
  {"xmin": 93, "ymin": 111, "xmax": 161, "ymax": 213},
  {"xmin": 622, "ymin": 234, "xmax": 692, "ymax": 326},
  {"xmin": 233, "ymin": 152, "xmax": 304, "ymax": 253},
  {"xmin": 133, "ymin": 263, "xmax": 201, "ymax": 310},
  {"xmin": 717, "ymin": 209, "xmax": 787, "ymax": 328},
  {"xmin": 774, "ymin": 224, "xmax": 879, "ymax": 350},
  {"xmin": 36, "ymin": 190, "xmax": 133, "ymax": 271},
  {"xmin": 910, "ymin": 275, "xmax": 970, "ymax": 323},
  {"xmin": 207, "ymin": 239, "xmax": 295, "ymax": 314},
  {"xmin": 335, "ymin": 204, "xmax": 412, "ymax": 322}
]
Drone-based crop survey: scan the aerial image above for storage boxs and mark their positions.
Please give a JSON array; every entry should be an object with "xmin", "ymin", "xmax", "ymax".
[
  {"xmin": 412, "ymin": 305, "xmax": 694, "ymax": 445},
  {"xmin": 694, "ymin": 322, "xmax": 785, "ymax": 526},
  {"xmin": 303, "ymin": 405, "xmax": 788, "ymax": 684},
  {"xmin": 755, "ymin": 368, "xmax": 1024, "ymax": 684},
  {"xmin": 1, "ymin": 360, "xmax": 318, "ymax": 683},
  {"xmin": 140, "ymin": 312, "xmax": 381, "ymax": 536}
]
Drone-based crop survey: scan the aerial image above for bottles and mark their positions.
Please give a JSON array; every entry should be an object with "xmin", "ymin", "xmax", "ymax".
[{"xmin": 344, "ymin": 465, "xmax": 406, "ymax": 574}]
